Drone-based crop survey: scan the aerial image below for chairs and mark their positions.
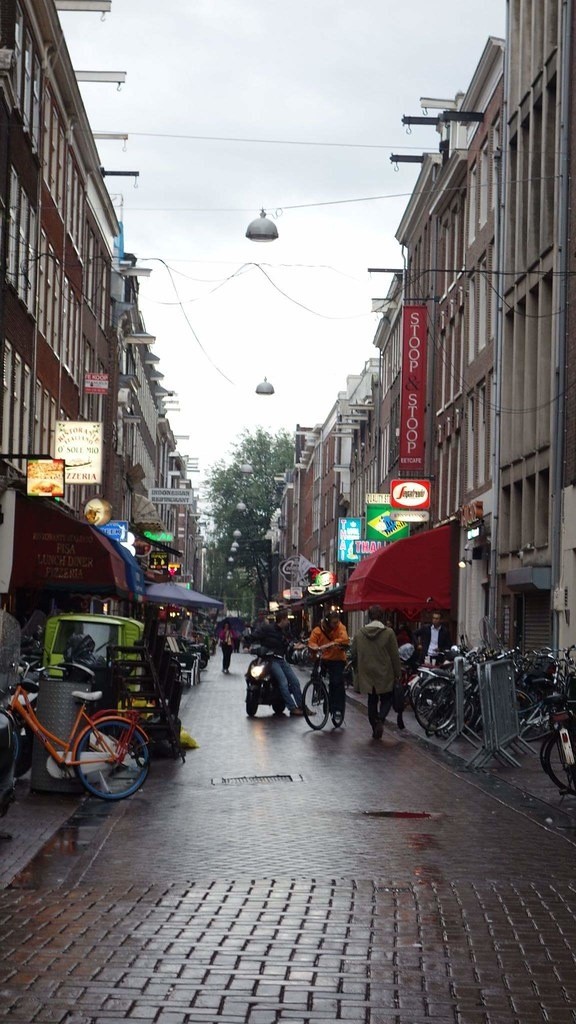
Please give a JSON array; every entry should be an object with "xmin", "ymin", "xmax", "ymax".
[{"xmin": 179, "ymin": 652, "xmax": 201, "ymax": 686}]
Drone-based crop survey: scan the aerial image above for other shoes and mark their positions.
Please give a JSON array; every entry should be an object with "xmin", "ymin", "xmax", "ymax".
[
  {"xmin": 222, "ymin": 668, "xmax": 228, "ymax": 673},
  {"xmin": 372, "ymin": 721, "xmax": 383, "ymax": 739},
  {"xmin": 298, "ymin": 705, "xmax": 317, "ymax": 716},
  {"xmin": 290, "ymin": 708, "xmax": 304, "ymax": 716}
]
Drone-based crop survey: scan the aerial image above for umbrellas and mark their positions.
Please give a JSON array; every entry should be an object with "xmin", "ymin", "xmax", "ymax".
[
  {"xmin": 216, "ymin": 617, "xmax": 246, "ymax": 633},
  {"xmin": 145, "ymin": 581, "xmax": 223, "ymax": 635}
]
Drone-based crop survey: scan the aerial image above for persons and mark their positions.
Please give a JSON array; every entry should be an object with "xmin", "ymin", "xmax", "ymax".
[
  {"xmin": 414, "ymin": 611, "xmax": 451, "ymax": 666},
  {"xmin": 351, "ymin": 606, "xmax": 400, "ymax": 740},
  {"xmin": 0, "ymin": 597, "xmax": 21, "ymax": 841},
  {"xmin": 243, "ymin": 610, "xmax": 317, "ymax": 716},
  {"xmin": 308, "ymin": 610, "xmax": 349, "ymax": 715},
  {"xmin": 218, "ymin": 623, "xmax": 239, "ymax": 674},
  {"xmin": 386, "ymin": 618, "xmax": 412, "ymax": 646},
  {"xmin": 234, "ymin": 635, "xmax": 240, "ymax": 653}
]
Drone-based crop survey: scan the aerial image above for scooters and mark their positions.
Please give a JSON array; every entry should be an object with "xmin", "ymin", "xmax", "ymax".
[{"xmin": 245, "ymin": 641, "xmax": 290, "ymax": 717}]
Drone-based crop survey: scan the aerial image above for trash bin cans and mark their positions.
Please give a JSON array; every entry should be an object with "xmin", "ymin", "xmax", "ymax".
[{"xmin": 30, "ymin": 661, "xmax": 96, "ymax": 797}]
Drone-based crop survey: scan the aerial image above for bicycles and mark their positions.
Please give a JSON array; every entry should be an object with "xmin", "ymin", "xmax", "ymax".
[
  {"xmin": 0, "ymin": 654, "xmax": 151, "ymax": 803},
  {"xmin": 292, "ymin": 629, "xmax": 353, "ymax": 688},
  {"xmin": 399, "ymin": 631, "xmax": 576, "ymax": 799},
  {"xmin": 299, "ymin": 638, "xmax": 352, "ymax": 731}
]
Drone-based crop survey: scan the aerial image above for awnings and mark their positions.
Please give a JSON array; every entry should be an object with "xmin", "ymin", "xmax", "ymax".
[
  {"xmin": 342, "ymin": 525, "xmax": 452, "ymax": 613},
  {"xmin": 10, "ymin": 497, "xmax": 145, "ymax": 595},
  {"xmin": 131, "ymin": 493, "xmax": 166, "ymax": 531}
]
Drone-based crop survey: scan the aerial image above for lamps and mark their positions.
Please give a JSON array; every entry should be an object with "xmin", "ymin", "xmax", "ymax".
[
  {"xmin": 458, "ymin": 557, "xmax": 472, "ymax": 569},
  {"xmin": 246, "ymin": 210, "xmax": 278, "ymax": 242},
  {"xmin": 228, "ymin": 541, "xmax": 239, "ymax": 562},
  {"xmin": 240, "ymin": 464, "xmax": 253, "ymax": 474},
  {"xmin": 236, "ymin": 503, "xmax": 246, "ymax": 512},
  {"xmin": 255, "ymin": 377, "xmax": 275, "ymax": 395},
  {"xmin": 233, "ymin": 529, "xmax": 242, "ymax": 538}
]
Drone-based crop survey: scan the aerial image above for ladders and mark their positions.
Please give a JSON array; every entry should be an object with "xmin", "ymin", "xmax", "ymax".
[{"xmin": 104, "ymin": 645, "xmax": 187, "ymax": 764}]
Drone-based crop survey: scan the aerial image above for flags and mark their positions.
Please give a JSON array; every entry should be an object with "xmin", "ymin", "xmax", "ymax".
[{"xmin": 299, "ymin": 556, "xmax": 315, "ymax": 575}]
